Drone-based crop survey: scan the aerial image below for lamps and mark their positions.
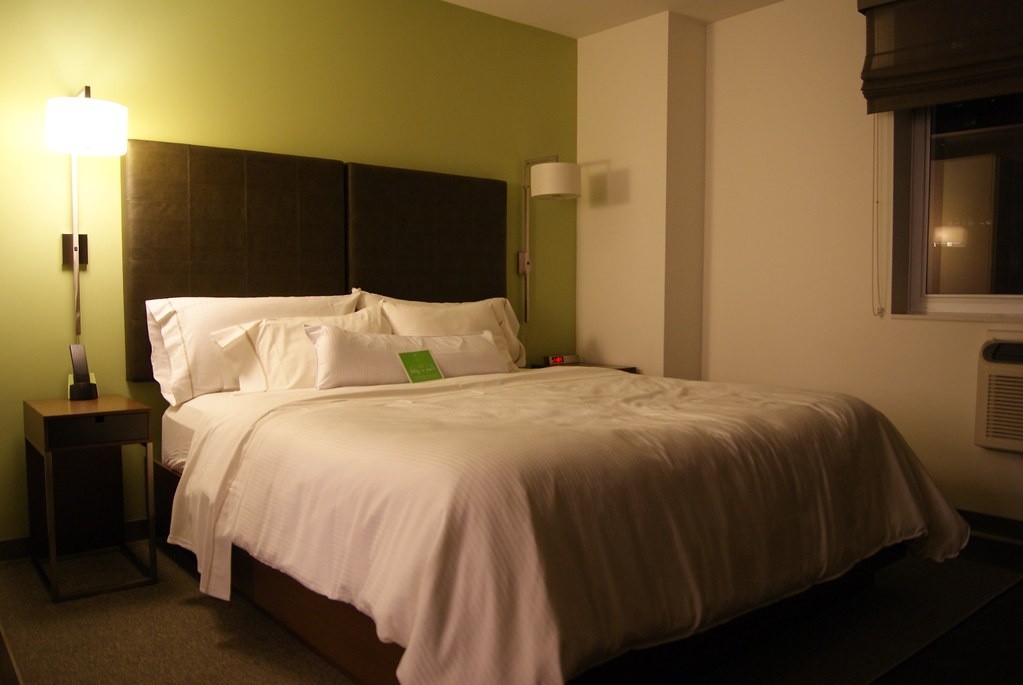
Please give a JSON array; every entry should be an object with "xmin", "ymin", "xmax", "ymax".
[
  {"xmin": 516, "ymin": 156, "xmax": 580, "ymax": 323},
  {"xmin": 47, "ymin": 85, "xmax": 130, "ymax": 336}
]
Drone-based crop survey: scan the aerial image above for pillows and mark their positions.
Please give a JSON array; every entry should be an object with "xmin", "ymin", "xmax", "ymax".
[
  {"xmin": 144, "ymin": 288, "xmax": 365, "ymax": 408},
  {"xmin": 303, "ymin": 322, "xmax": 517, "ymax": 389},
  {"xmin": 350, "ymin": 287, "xmax": 527, "ymax": 367},
  {"xmin": 381, "ymin": 300, "xmax": 508, "ymax": 352},
  {"xmin": 208, "ymin": 302, "xmax": 390, "ymax": 397}
]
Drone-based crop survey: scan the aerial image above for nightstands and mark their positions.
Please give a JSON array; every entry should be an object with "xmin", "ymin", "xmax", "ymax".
[
  {"xmin": 529, "ymin": 365, "xmax": 638, "ymax": 374},
  {"xmin": 22, "ymin": 399, "xmax": 159, "ymax": 601}
]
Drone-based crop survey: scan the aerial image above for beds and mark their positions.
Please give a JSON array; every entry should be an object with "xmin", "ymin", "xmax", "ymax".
[{"xmin": 148, "ymin": 384, "xmax": 968, "ymax": 685}]
